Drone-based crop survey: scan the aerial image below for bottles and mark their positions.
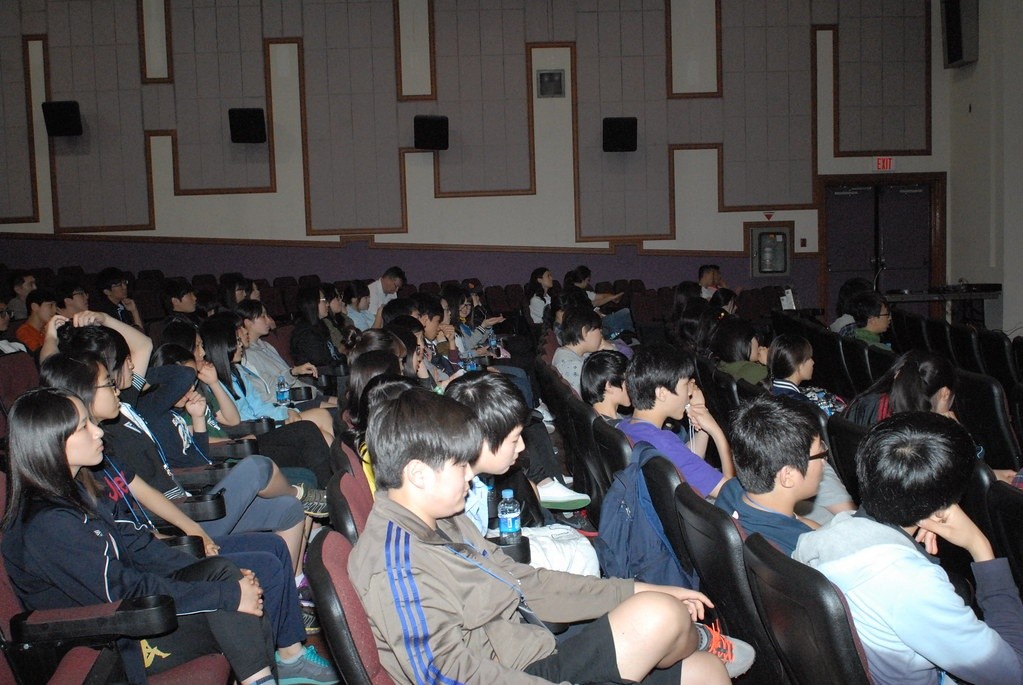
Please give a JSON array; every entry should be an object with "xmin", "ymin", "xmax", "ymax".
[
  {"xmin": 497, "ymin": 490, "xmax": 521, "ymax": 545},
  {"xmin": 488, "ymin": 330, "xmax": 496, "ymax": 349},
  {"xmin": 816, "ymin": 392, "xmax": 832, "ymax": 417},
  {"xmin": 465, "ymin": 352, "xmax": 477, "ymax": 371},
  {"xmin": 275, "ymin": 376, "xmax": 291, "ymax": 405},
  {"xmin": 608, "ymin": 328, "xmax": 624, "ymax": 340}
]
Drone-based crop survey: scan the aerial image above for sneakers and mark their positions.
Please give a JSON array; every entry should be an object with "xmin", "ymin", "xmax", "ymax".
[
  {"xmin": 553, "ymin": 507, "xmax": 600, "ymax": 537},
  {"xmin": 300, "ymin": 611, "xmax": 321, "ymax": 635},
  {"xmin": 275, "ymin": 642, "xmax": 341, "ymax": 685},
  {"xmin": 293, "ymin": 482, "xmax": 333, "ymax": 518},
  {"xmin": 692, "ymin": 621, "xmax": 757, "ymax": 679},
  {"xmin": 295, "ymin": 576, "xmax": 315, "ymax": 608}
]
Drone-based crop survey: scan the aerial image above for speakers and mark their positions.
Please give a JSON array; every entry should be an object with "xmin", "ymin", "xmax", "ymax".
[
  {"xmin": 228, "ymin": 107, "xmax": 267, "ymax": 143},
  {"xmin": 41, "ymin": 99, "xmax": 82, "ymax": 136},
  {"xmin": 603, "ymin": 116, "xmax": 637, "ymax": 153},
  {"xmin": 414, "ymin": 113, "xmax": 449, "ymax": 151}
]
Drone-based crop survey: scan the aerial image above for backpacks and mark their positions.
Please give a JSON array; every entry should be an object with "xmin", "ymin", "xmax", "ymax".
[{"xmin": 592, "ymin": 443, "xmax": 693, "ymax": 590}]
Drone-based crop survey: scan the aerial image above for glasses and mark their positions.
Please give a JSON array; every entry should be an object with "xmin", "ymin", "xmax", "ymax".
[
  {"xmin": 809, "ymin": 439, "xmax": 829, "ymax": 462},
  {"xmin": 93, "ymin": 378, "xmax": 117, "ymax": 394},
  {"xmin": 414, "ymin": 344, "xmax": 421, "ymax": 356},
  {"xmin": 0, "ymin": 310, "xmax": 14, "ymax": 319},
  {"xmin": 462, "ymin": 304, "xmax": 472, "ymax": 310},
  {"xmin": 875, "ymin": 311, "xmax": 892, "ymax": 320},
  {"xmin": 111, "ymin": 279, "xmax": 129, "ymax": 289},
  {"xmin": 71, "ymin": 291, "xmax": 90, "ymax": 300}
]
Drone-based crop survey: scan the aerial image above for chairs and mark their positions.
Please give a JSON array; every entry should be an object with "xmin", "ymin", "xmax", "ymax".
[{"xmin": 1, "ymin": 266, "xmax": 1023, "ymax": 685}]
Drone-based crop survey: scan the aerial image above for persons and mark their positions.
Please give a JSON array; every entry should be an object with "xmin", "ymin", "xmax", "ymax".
[{"xmin": 0, "ymin": 263, "xmax": 1023, "ymax": 685}]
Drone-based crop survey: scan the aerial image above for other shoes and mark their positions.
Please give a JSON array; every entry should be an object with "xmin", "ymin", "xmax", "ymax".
[
  {"xmin": 544, "ymin": 424, "xmax": 556, "ymax": 435},
  {"xmin": 535, "ymin": 476, "xmax": 591, "ymax": 510},
  {"xmin": 535, "ymin": 406, "xmax": 556, "ymax": 421},
  {"xmin": 538, "ymin": 398, "xmax": 550, "ymax": 412}
]
